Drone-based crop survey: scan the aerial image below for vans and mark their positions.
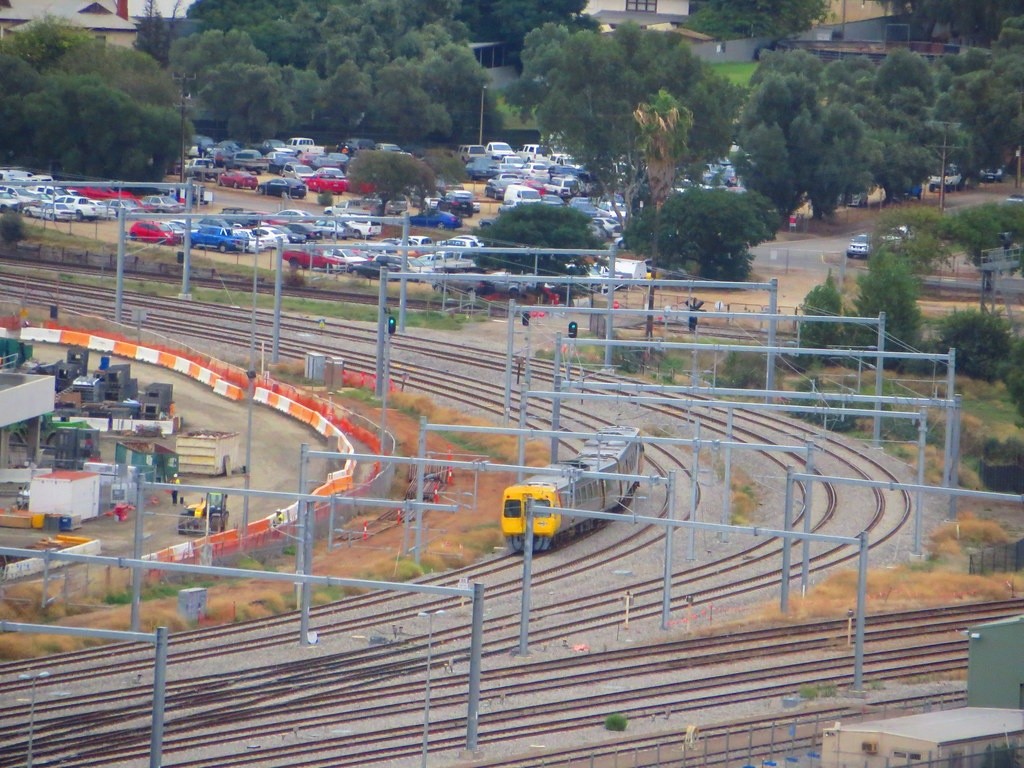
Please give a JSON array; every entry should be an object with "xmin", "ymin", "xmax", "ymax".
[{"xmin": 338, "ymin": 138, "xmax": 376, "ymax": 156}]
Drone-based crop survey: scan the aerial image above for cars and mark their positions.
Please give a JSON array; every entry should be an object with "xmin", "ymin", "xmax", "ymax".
[
  {"xmin": 128, "ymin": 199, "xmax": 382, "ymax": 252},
  {"xmin": 282, "ymin": 235, "xmax": 485, "ymax": 277},
  {"xmin": 579, "ymin": 191, "xmax": 624, "ymax": 237},
  {"xmin": 363, "ymin": 184, "xmax": 480, "ymax": 230},
  {"xmin": 375, "ymin": 143, "xmax": 412, "ymax": 157},
  {"xmin": 187, "ymin": 137, "xmax": 349, "ymax": 198},
  {"xmin": 0, "ymin": 169, "xmax": 185, "ymax": 221},
  {"xmin": 459, "ymin": 142, "xmax": 597, "ymax": 207},
  {"xmin": 930, "ymin": 162, "xmax": 962, "ymax": 194}
]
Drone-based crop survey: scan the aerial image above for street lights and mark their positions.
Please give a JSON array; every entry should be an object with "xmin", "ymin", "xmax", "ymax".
[{"xmin": 479, "ymin": 82, "xmax": 487, "ymax": 143}]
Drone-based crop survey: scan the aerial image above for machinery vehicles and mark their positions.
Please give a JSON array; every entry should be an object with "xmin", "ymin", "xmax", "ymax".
[{"xmin": 178, "ymin": 491, "xmax": 229, "ymax": 538}]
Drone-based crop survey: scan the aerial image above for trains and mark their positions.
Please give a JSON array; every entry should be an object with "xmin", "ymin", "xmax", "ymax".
[{"xmin": 501, "ymin": 427, "xmax": 645, "ymax": 555}]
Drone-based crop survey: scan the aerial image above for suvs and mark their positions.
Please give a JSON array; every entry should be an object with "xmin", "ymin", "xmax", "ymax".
[{"xmin": 847, "ymin": 234, "xmax": 870, "ymax": 257}]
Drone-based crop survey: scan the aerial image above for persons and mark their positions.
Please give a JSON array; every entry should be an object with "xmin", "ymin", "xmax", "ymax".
[
  {"xmin": 170, "ymin": 473, "xmax": 180, "ymax": 505},
  {"xmin": 197, "ymin": 137, "xmax": 208, "ymax": 158},
  {"xmin": 336, "ymin": 141, "xmax": 353, "ymax": 156}
]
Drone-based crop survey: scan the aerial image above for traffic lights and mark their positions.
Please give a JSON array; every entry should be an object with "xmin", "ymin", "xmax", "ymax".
[
  {"xmin": 568, "ymin": 321, "xmax": 578, "ymax": 338},
  {"xmin": 389, "ymin": 316, "xmax": 395, "ymax": 336}
]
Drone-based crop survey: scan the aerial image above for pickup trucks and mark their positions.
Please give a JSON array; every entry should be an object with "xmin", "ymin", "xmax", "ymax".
[
  {"xmin": 187, "ymin": 157, "xmax": 219, "ymax": 180},
  {"xmin": 190, "ymin": 224, "xmax": 248, "ymax": 253},
  {"xmin": 53, "ymin": 195, "xmax": 107, "ymax": 220}
]
